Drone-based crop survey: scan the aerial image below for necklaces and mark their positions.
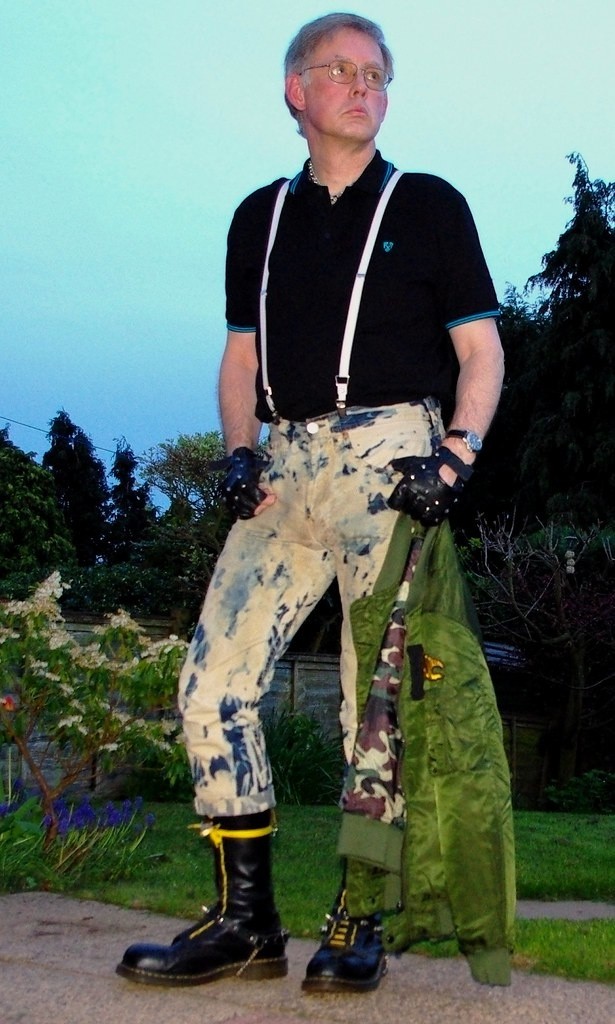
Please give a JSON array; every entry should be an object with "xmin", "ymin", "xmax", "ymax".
[{"xmin": 308, "ymin": 160, "xmax": 346, "ymax": 205}]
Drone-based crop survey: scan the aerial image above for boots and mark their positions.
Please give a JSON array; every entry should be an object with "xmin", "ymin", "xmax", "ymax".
[
  {"xmin": 114, "ymin": 810, "xmax": 289, "ymax": 987},
  {"xmin": 301, "ymin": 856, "xmax": 388, "ymax": 992}
]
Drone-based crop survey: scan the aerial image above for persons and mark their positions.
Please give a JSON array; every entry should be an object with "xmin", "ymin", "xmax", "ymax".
[{"xmin": 115, "ymin": 12, "xmax": 504, "ymax": 993}]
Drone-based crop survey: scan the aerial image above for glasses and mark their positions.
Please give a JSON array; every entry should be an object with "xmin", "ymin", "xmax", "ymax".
[{"xmin": 298, "ymin": 59, "xmax": 394, "ymax": 92}]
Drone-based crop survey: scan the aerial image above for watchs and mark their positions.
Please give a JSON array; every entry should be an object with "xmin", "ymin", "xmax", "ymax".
[{"xmin": 445, "ymin": 428, "xmax": 483, "ymax": 454}]
